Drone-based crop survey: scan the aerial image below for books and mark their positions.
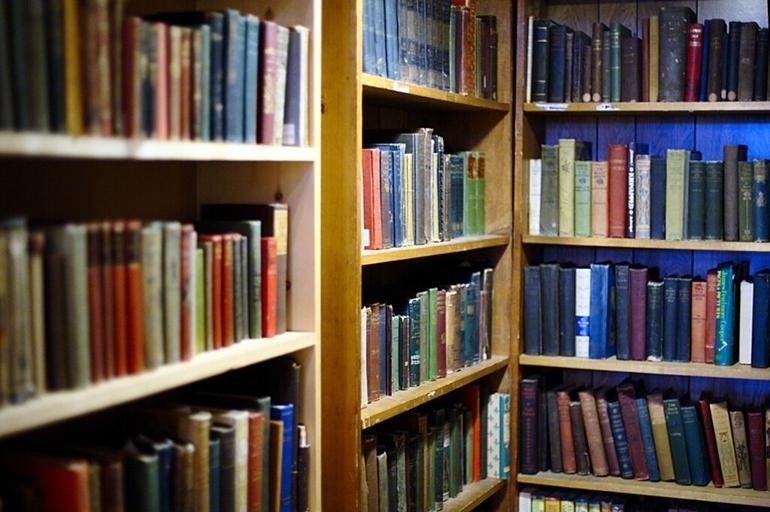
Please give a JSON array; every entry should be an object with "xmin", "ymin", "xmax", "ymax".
[
  {"xmin": 529, "ymin": 137, "xmax": 770, "ymax": 242},
  {"xmin": 519, "ymin": 262, "xmax": 770, "ymax": 511},
  {"xmin": 359, "ymin": 1, "xmax": 509, "ymax": 511},
  {"xmin": 3, "ymin": 2, "xmax": 312, "ymax": 512},
  {"xmin": 525, "ymin": 6, "xmax": 770, "ymax": 103}
]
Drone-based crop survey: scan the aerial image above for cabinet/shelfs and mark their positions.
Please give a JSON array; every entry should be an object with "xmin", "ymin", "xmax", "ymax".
[
  {"xmin": 0, "ymin": 1, "xmax": 323, "ymax": 512},
  {"xmin": 518, "ymin": 0, "xmax": 770, "ymax": 512},
  {"xmin": 323, "ymin": 1, "xmax": 518, "ymax": 511}
]
